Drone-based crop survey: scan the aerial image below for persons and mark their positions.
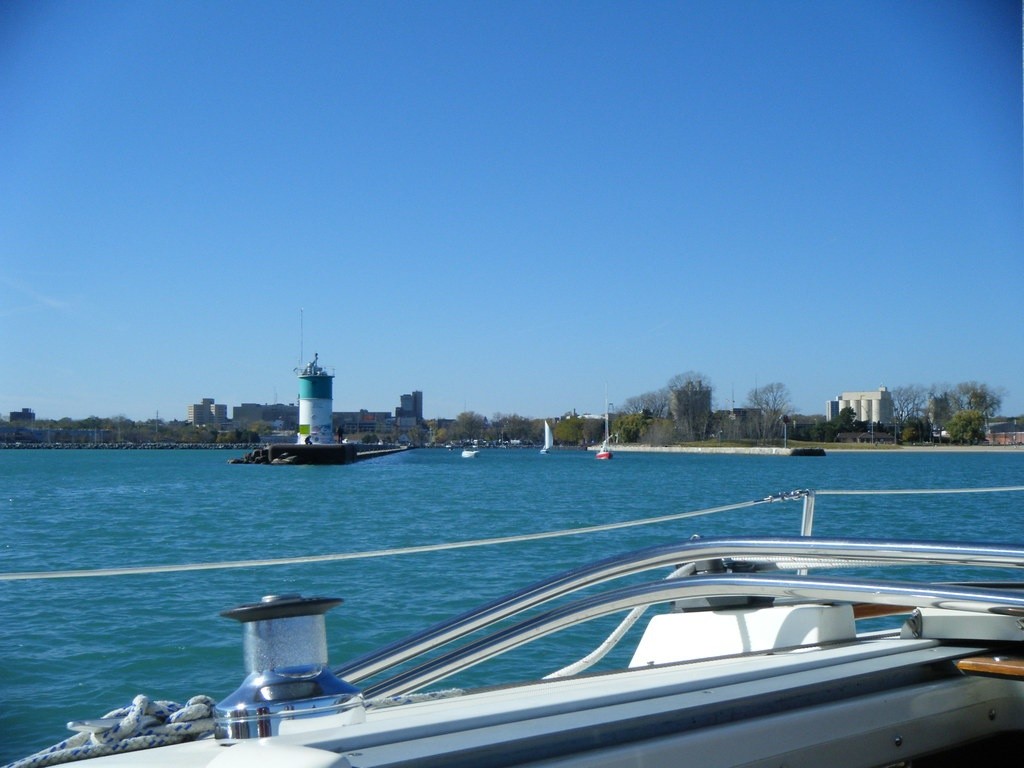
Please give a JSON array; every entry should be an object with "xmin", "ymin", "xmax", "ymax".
[
  {"xmin": 304, "ymin": 435, "xmax": 312, "ymax": 445},
  {"xmin": 336, "ymin": 424, "xmax": 343, "ymax": 444}
]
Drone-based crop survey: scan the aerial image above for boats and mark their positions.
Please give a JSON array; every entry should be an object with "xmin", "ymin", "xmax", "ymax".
[{"xmin": 462, "ymin": 450, "xmax": 480, "ymax": 458}]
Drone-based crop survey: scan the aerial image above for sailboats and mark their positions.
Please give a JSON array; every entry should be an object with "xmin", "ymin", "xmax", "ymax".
[
  {"xmin": 595, "ymin": 382, "xmax": 613, "ymax": 459},
  {"xmin": 540, "ymin": 420, "xmax": 553, "ymax": 454}
]
